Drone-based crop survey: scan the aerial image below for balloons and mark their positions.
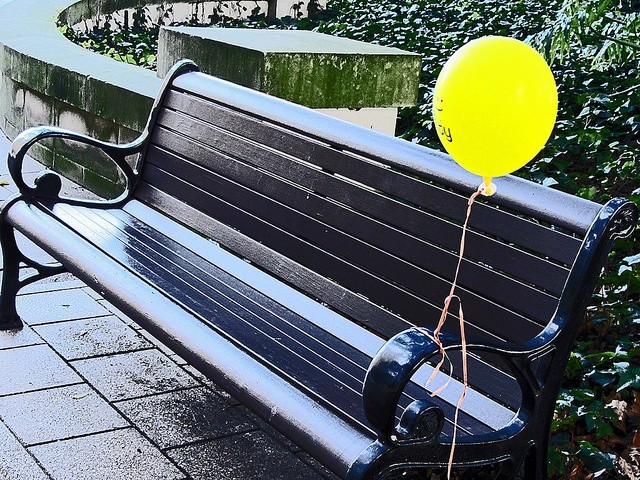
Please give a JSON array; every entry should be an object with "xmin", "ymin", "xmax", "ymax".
[{"xmin": 430, "ymin": 34, "xmax": 559, "ymax": 196}]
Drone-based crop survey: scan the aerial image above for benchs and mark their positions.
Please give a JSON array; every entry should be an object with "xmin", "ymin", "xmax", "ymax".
[{"xmin": 0, "ymin": 60, "xmax": 638, "ymax": 479}]
